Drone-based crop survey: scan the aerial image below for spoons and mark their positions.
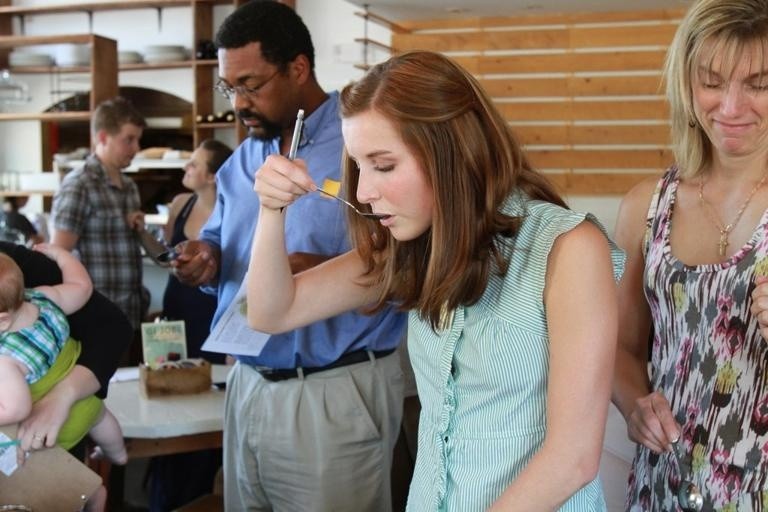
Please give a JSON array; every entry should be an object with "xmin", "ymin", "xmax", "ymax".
[
  {"xmin": 156, "ymin": 249, "xmax": 187, "ymax": 265},
  {"xmin": 316, "ymin": 186, "xmax": 391, "ymax": 221},
  {"xmin": 671, "ymin": 441, "xmax": 704, "ymax": 512}
]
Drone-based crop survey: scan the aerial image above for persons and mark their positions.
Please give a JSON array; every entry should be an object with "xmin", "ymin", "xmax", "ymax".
[
  {"xmin": 605, "ymin": 2, "xmax": 766, "ymax": 512},
  {"xmin": 164, "ymin": 1, "xmax": 414, "ymax": 512},
  {"xmin": 244, "ymin": 50, "xmax": 627, "ymax": 510},
  {"xmin": 0, "ymin": 205, "xmax": 136, "ymax": 512},
  {"xmin": 48, "ymin": 95, "xmax": 153, "ymax": 363},
  {"xmin": 125, "ymin": 137, "xmax": 239, "ymax": 509}
]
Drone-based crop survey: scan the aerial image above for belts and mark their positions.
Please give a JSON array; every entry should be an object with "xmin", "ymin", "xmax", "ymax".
[{"xmin": 250, "ymin": 346, "xmax": 396, "ymax": 383}]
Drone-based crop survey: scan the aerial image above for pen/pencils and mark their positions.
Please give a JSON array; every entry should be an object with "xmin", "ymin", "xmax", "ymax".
[
  {"xmin": 279, "ymin": 109, "xmax": 304, "ymax": 216},
  {"xmin": 0, "ymin": 439, "xmax": 22, "ymax": 447}
]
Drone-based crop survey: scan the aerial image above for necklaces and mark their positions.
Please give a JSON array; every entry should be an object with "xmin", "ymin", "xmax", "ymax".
[{"xmin": 694, "ymin": 166, "xmax": 766, "ymax": 260}]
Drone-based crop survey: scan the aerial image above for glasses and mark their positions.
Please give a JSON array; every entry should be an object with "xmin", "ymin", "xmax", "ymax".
[{"xmin": 214, "ymin": 70, "xmax": 280, "ymax": 99}]
